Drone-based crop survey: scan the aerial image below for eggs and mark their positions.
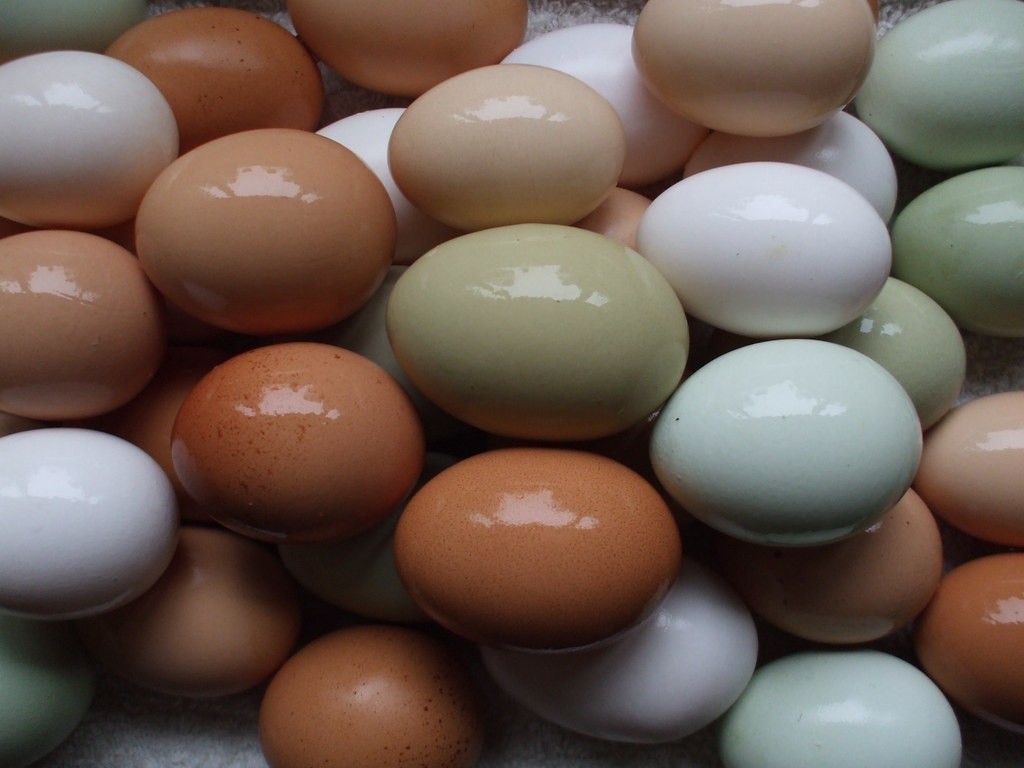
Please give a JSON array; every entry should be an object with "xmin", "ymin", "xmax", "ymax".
[{"xmin": 0, "ymin": 0, "xmax": 1024, "ymax": 768}]
